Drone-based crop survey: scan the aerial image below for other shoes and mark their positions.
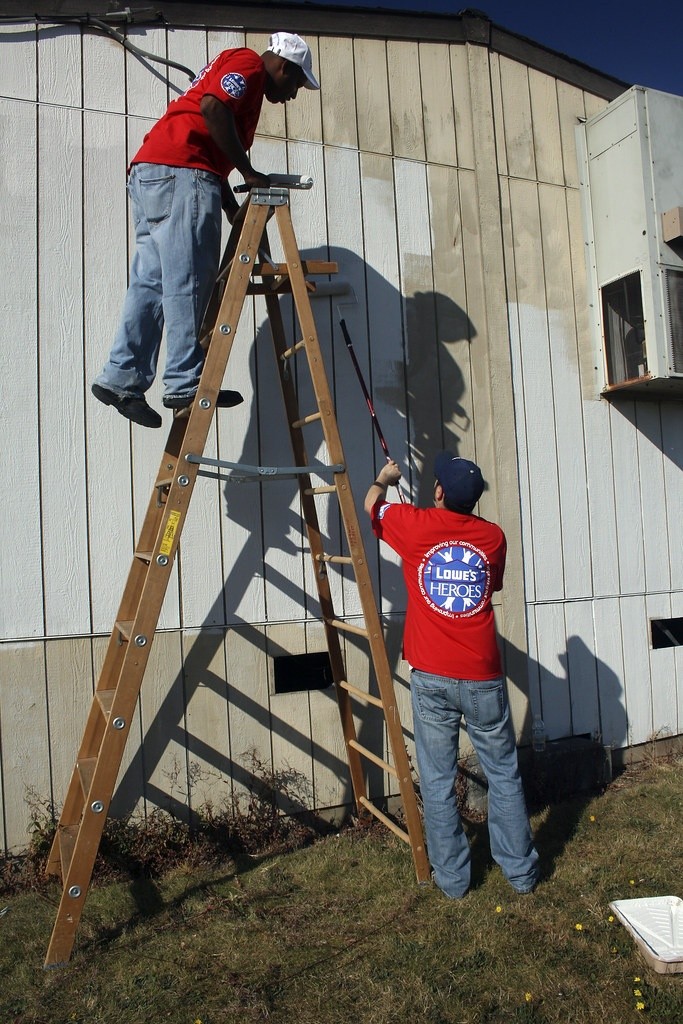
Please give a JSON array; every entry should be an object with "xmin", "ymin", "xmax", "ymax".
[
  {"xmin": 162, "ymin": 390, "xmax": 244, "ymax": 409},
  {"xmin": 91, "ymin": 384, "xmax": 162, "ymax": 428}
]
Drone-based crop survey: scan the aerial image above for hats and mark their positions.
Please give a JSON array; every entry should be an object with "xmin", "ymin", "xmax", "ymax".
[
  {"xmin": 433, "ymin": 455, "xmax": 485, "ymax": 510},
  {"xmin": 266, "ymin": 32, "xmax": 321, "ymax": 90}
]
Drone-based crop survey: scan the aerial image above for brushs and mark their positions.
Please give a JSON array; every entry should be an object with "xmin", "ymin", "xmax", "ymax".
[
  {"xmin": 307, "ymin": 275, "xmax": 411, "ymax": 508},
  {"xmin": 233, "ymin": 171, "xmax": 314, "ymax": 197}
]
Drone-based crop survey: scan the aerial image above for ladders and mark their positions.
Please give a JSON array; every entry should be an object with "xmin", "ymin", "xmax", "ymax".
[{"xmin": 43, "ymin": 187, "xmax": 434, "ymax": 971}]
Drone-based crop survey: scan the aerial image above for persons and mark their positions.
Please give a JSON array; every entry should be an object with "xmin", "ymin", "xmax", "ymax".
[
  {"xmin": 363, "ymin": 456, "xmax": 543, "ymax": 895},
  {"xmin": 90, "ymin": 31, "xmax": 320, "ymax": 428}
]
